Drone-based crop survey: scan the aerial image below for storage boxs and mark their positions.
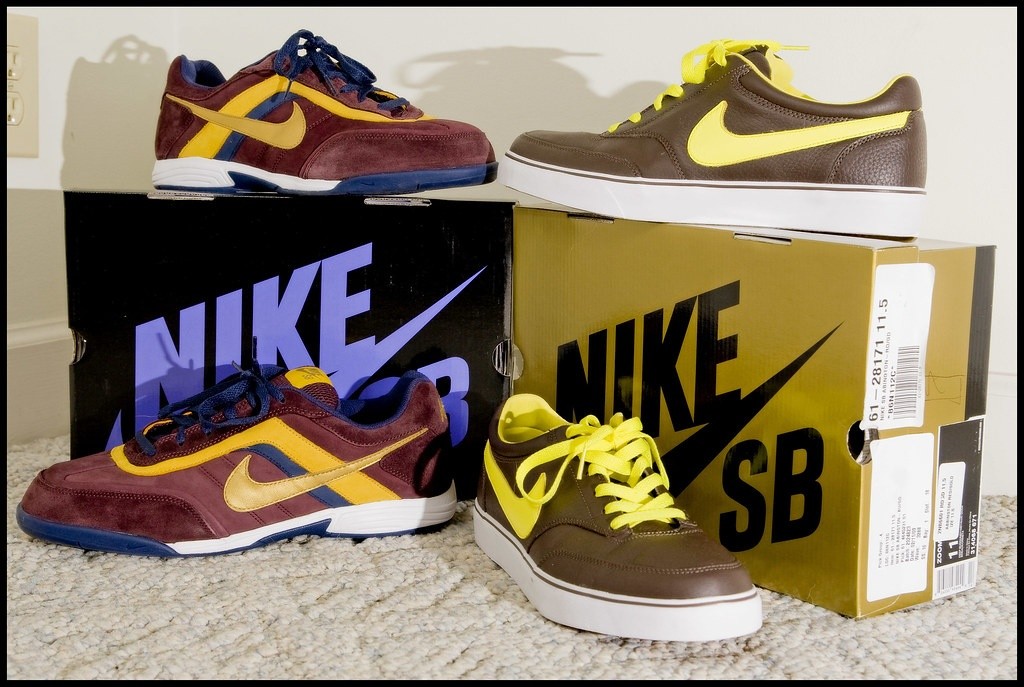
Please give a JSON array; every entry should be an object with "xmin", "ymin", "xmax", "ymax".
[
  {"xmin": 62, "ymin": 189, "xmax": 520, "ymax": 502},
  {"xmin": 512, "ymin": 201, "xmax": 996, "ymax": 619}
]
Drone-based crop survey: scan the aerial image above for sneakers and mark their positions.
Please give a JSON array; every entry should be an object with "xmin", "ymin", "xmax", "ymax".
[
  {"xmin": 150, "ymin": 29, "xmax": 500, "ymax": 194},
  {"xmin": 469, "ymin": 394, "xmax": 764, "ymax": 642},
  {"xmin": 503, "ymin": 37, "xmax": 928, "ymax": 239},
  {"xmin": 15, "ymin": 358, "xmax": 457, "ymax": 557}
]
